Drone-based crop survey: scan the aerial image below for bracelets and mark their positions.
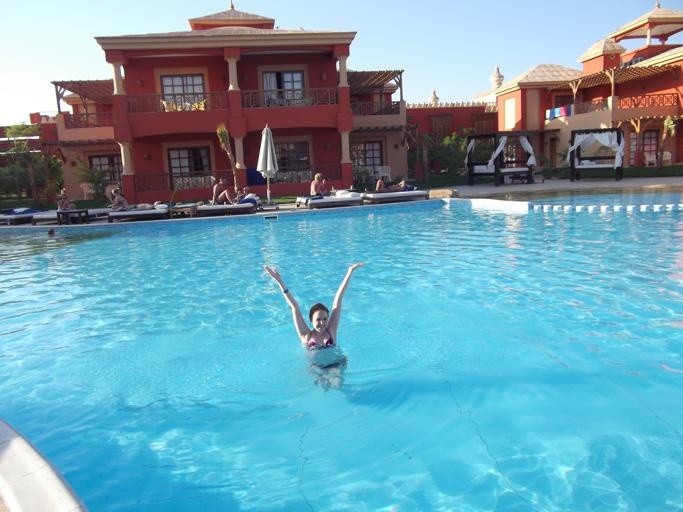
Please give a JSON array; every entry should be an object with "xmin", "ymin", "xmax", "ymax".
[{"xmin": 282, "ymin": 288, "xmax": 288, "ymax": 294}]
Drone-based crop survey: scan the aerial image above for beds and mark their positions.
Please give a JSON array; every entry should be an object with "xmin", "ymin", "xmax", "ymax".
[
  {"xmin": 570, "ymin": 128, "xmax": 623, "ymax": 181},
  {"xmin": 465, "ymin": 131, "xmax": 533, "ymax": 186},
  {"xmin": 0, "ymin": 166, "xmax": 430, "ymax": 226}
]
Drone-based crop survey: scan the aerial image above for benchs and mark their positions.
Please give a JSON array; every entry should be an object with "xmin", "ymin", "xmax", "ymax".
[{"xmin": 508, "ymin": 175, "xmax": 544, "ymax": 183}]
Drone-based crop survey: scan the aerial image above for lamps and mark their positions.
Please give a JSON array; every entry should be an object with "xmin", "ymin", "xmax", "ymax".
[
  {"xmin": 136, "ymin": 79, "xmax": 144, "ymax": 87},
  {"xmin": 324, "ymin": 143, "xmax": 331, "ymax": 150},
  {"xmin": 144, "ymin": 153, "xmax": 151, "ymax": 160},
  {"xmin": 320, "ymin": 72, "xmax": 327, "ymax": 80}
]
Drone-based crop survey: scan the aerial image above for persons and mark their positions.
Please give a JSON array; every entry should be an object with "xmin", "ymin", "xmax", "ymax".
[
  {"xmin": 364, "ymin": 179, "xmax": 405, "ymax": 193},
  {"xmin": 211, "ymin": 176, "xmax": 234, "ymax": 204},
  {"xmin": 319, "ymin": 177, "xmax": 329, "ymax": 196},
  {"xmin": 307, "ymin": 173, "xmax": 322, "ymax": 199},
  {"xmin": 47, "ymin": 228, "xmax": 56, "ymax": 237},
  {"xmin": 207, "ymin": 185, "xmax": 249, "ymax": 204},
  {"xmin": 56, "ymin": 194, "xmax": 70, "ymax": 224},
  {"xmin": 111, "ymin": 188, "xmax": 128, "ymax": 220},
  {"xmin": 265, "ymin": 261, "xmax": 366, "ymax": 392}
]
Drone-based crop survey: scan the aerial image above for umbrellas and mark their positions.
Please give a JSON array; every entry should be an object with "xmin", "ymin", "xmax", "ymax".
[{"xmin": 254, "ymin": 123, "xmax": 278, "ymax": 205}]
